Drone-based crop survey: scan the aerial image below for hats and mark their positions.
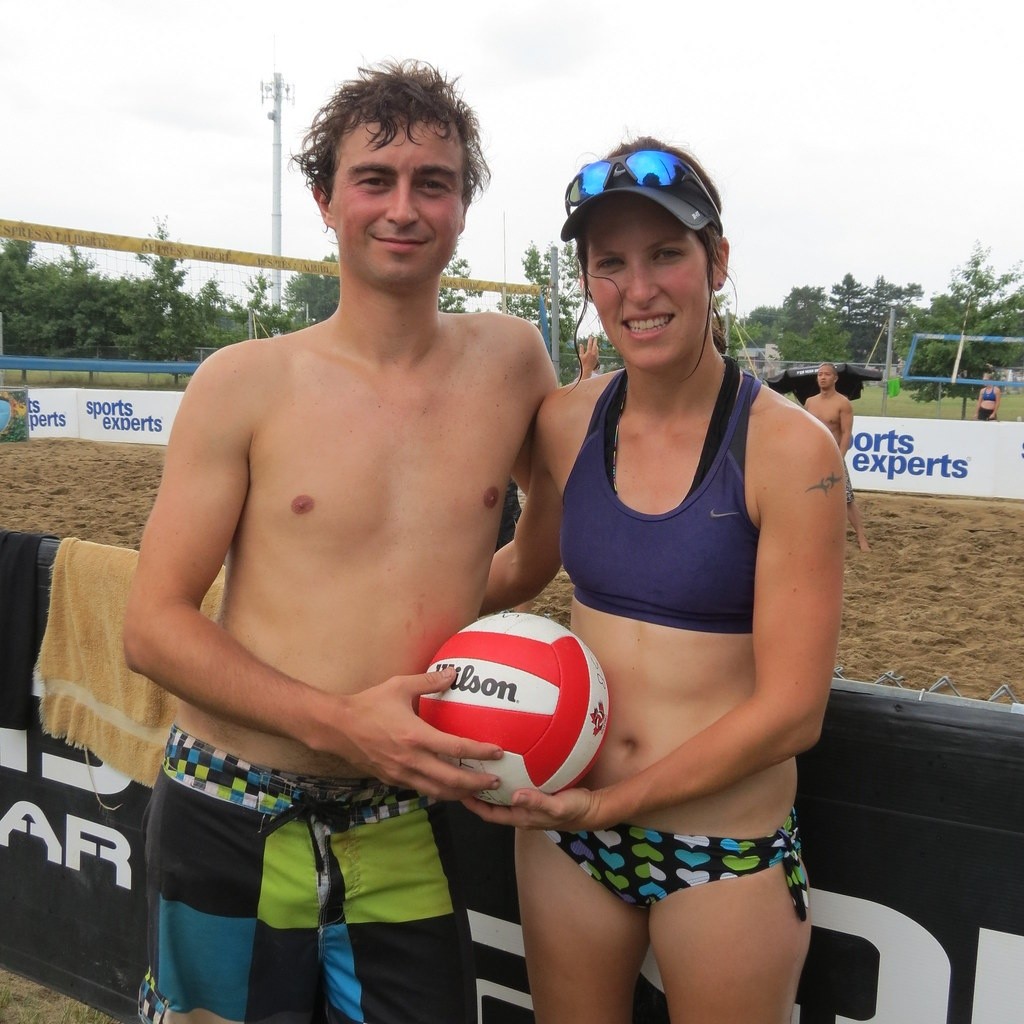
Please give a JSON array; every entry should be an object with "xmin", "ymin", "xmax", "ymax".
[{"xmin": 559, "ymin": 165, "xmax": 717, "ymax": 242}]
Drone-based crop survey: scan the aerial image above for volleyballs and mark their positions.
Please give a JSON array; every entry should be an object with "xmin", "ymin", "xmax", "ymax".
[{"xmin": 418, "ymin": 608, "xmax": 610, "ymax": 803}]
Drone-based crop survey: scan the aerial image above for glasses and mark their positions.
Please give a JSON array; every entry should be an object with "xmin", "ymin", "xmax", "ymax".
[{"xmin": 564, "ymin": 148, "xmax": 721, "ymax": 215}]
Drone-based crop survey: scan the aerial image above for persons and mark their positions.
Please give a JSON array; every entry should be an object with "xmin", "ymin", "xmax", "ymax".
[
  {"xmin": 120, "ymin": 69, "xmax": 605, "ymax": 1024},
  {"xmin": 974, "ymin": 373, "xmax": 1001, "ymax": 421},
  {"xmin": 481, "ymin": 136, "xmax": 847, "ymax": 1024},
  {"xmin": 803, "ymin": 364, "xmax": 869, "ymax": 553}
]
